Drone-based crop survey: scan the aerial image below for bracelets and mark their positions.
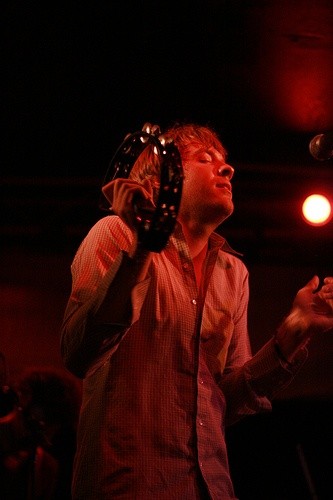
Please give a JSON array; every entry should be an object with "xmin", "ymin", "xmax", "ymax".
[{"xmin": 274, "ymin": 342, "xmax": 299, "ymax": 366}]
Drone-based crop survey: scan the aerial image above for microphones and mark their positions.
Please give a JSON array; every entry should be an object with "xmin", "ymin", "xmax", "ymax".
[{"xmin": 309, "ymin": 130, "xmax": 333, "ymax": 160}]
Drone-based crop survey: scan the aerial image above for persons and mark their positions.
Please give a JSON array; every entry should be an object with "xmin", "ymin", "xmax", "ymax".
[
  {"xmin": 59, "ymin": 125, "xmax": 333, "ymax": 500},
  {"xmin": 0, "ymin": 354, "xmax": 80, "ymax": 500}
]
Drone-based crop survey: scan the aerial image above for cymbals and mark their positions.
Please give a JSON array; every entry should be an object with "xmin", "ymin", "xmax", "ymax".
[{"xmin": 101, "ymin": 122, "xmax": 184, "ymax": 251}]
